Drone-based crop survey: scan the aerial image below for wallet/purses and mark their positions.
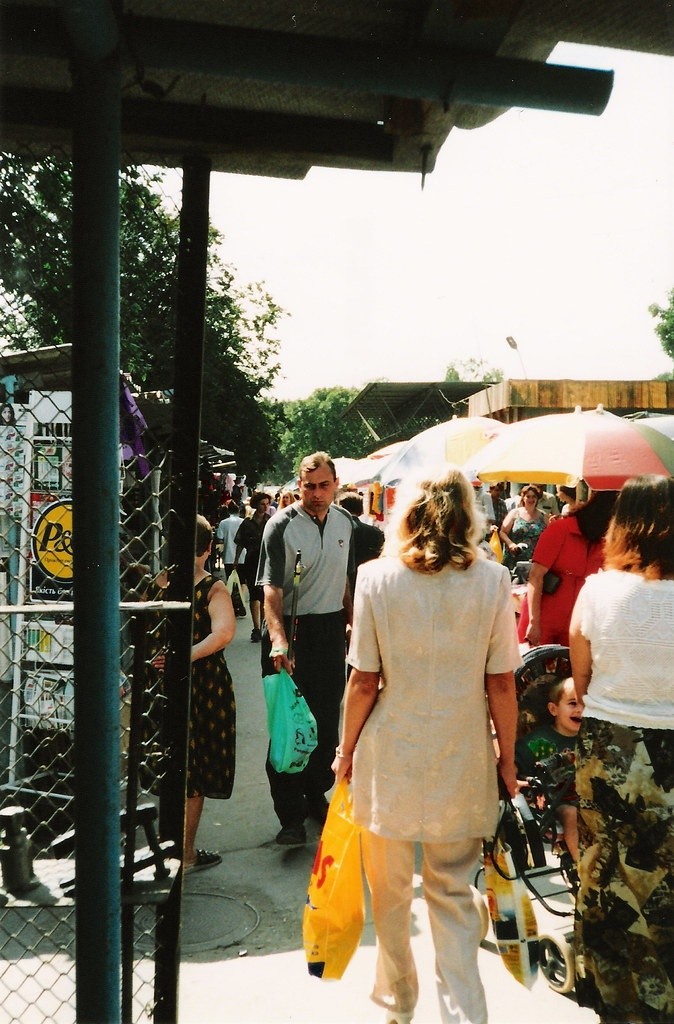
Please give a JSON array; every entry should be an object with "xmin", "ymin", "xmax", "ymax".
[{"xmin": 526, "ymin": 565, "xmax": 562, "ymax": 594}]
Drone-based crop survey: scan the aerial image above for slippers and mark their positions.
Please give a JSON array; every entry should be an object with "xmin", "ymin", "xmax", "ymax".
[{"xmin": 182, "ymin": 848, "xmax": 222, "ymax": 875}]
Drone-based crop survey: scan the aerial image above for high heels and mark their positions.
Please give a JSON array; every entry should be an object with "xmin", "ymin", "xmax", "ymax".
[{"xmin": 386, "ymin": 1005, "xmax": 413, "ymax": 1024}]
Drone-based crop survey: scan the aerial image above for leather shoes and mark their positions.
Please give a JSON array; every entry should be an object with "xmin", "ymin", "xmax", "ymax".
[
  {"xmin": 305, "ymin": 797, "xmax": 330, "ymax": 822},
  {"xmin": 277, "ymin": 824, "xmax": 306, "ymax": 844}
]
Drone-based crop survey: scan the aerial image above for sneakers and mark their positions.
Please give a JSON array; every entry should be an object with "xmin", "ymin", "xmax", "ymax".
[{"xmin": 250, "ymin": 629, "xmax": 262, "ymax": 642}]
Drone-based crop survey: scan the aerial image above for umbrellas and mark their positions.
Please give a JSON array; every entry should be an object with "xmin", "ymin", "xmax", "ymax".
[{"xmin": 283, "ymin": 403, "xmax": 674, "ymax": 490}]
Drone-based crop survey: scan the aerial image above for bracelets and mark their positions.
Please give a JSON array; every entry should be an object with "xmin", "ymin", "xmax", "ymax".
[{"xmin": 335, "ymin": 745, "xmax": 351, "ymax": 761}]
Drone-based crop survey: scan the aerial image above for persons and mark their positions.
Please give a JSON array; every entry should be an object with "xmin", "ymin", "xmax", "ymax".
[
  {"xmin": 218, "ymin": 468, "xmax": 300, "ymax": 643},
  {"xmin": 498, "ymin": 471, "xmax": 674, "ymax": 1024},
  {"xmin": 255, "ymin": 451, "xmax": 357, "ymax": 844},
  {"xmin": 141, "ymin": 514, "xmax": 235, "ymax": 876},
  {"xmin": 331, "ymin": 471, "xmax": 525, "ymax": 1024},
  {"xmin": 339, "ymin": 498, "xmax": 384, "ymax": 601}
]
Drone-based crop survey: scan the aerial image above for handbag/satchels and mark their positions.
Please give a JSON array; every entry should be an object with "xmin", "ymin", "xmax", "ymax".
[
  {"xmin": 489, "ymin": 529, "xmax": 504, "ymax": 565},
  {"xmin": 480, "ymin": 774, "xmax": 542, "ymax": 992},
  {"xmin": 301, "ymin": 777, "xmax": 368, "ymax": 980},
  {"xmin": 260, "ymin": 669, "xmax": 317, "ymax": 775},
  {"xmin": 225, "ymin": 569, "xmax": 246, "ymax": 618}
]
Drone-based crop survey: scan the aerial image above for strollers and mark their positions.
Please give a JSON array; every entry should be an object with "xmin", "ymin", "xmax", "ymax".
[{"xmin": 466, "ymin": 643, "xmax": 580, "ymax": 994}]
